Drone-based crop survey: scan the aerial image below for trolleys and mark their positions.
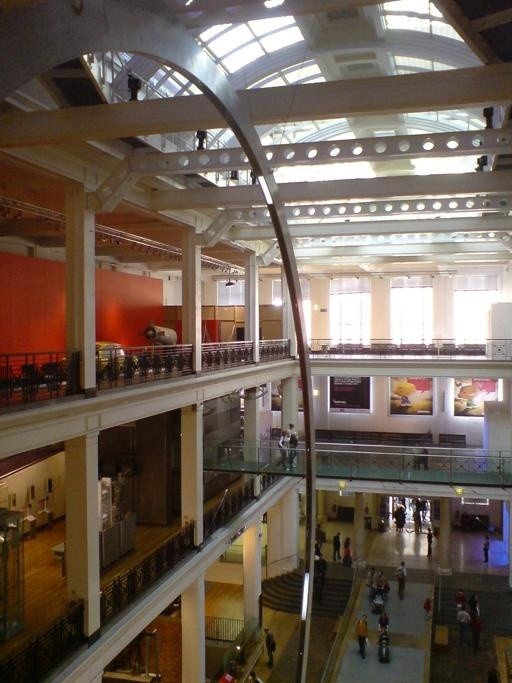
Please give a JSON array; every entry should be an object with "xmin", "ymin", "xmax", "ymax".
[
  {"xmin": 372, "ymin": 587, "xmax": 385, "ymax": 613},
  {"xmin": 378, "ymin": 631, "xmax": 390, "ymax": 661}
]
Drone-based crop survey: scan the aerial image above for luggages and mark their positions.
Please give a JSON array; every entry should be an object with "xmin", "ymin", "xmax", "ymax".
[{"xmin": 378, "ymin": 632, "xmax": 390, "ymax": 663}]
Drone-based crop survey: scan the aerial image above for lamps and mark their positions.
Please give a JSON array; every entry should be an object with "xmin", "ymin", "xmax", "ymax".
[{"xmin": 0, "ymin": 195, "xmax": 241, "ymax": 277}]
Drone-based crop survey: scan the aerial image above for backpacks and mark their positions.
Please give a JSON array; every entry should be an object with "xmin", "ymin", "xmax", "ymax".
[
  {"xmin": 396, "ymin": 567, "xmax": 405, "ymax": 582},
  {"xmin": 272, "ymin": 641, "xmax": 276, "ymax": 652}
]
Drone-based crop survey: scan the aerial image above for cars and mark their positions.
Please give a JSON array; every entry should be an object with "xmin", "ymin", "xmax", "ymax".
[{"xmin": 96, "ymin": 341, "xmax": 139, "ymax": 375}]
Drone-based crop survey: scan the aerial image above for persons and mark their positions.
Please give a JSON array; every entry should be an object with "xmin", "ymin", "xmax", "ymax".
[
  {"xmin": 249, "ymin": 671, "xmax": 263, "ymax": 683},
  {"xmin": 483, "ymin": 535, "xmax": 490, "ymax": 562},
  {"xmin": 355, "ymin": 614, "xmax": 368, "ymax": 658},
  {"xmin": 423, "ymin": 597, "xmax": 433, "ymax": 633},
  {"xmin": 278, "ymin": 424, "xmax": 299, "ymax": 471},
  {"xmin": 394, "ymin": 496, "xmax": 425, "ymax": 534},
  {"xmin": 314, "ymin": 523, "xmax": 327, "ymax": 601},
  {"xmin": 378, "ymin": 612, "xmax": 390, "ymax": 634},
  {"xmin": 427, "ymin": 528, "xmax": 433, "ymax": 556},
  {"xmin": 413, "ymin": 442, "xmax": 428, "ymax": 470},
  {"xmin": 394, "ymin": 562, "xmax": 407, "ymax": 599},
  {"xmin": 264, "ymin": 626, "xmax": 276, "ymax": 667},
  {"xmin": 367, "ymin": 567, "xmax": 386, "ymax": 602},
  {"xmin": 457, "ymin": 588, "xmax": 483, "ymax": 652},
  {"xmin": 333, "ymin": 532, "xmax": 352, "ymax": 567}
]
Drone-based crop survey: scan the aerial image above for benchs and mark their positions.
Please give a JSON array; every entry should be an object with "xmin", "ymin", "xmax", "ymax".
[
  {"xmin": 334, "ymin": 342, "xmax": 486, "ymax": 355},
  {"xmin": 439, "ymin": 434, "xmax": 466, "ymax": 447},
  {"xmin": 315, "ymin": 429, "xmax": 433, "ymax": 446}
]
